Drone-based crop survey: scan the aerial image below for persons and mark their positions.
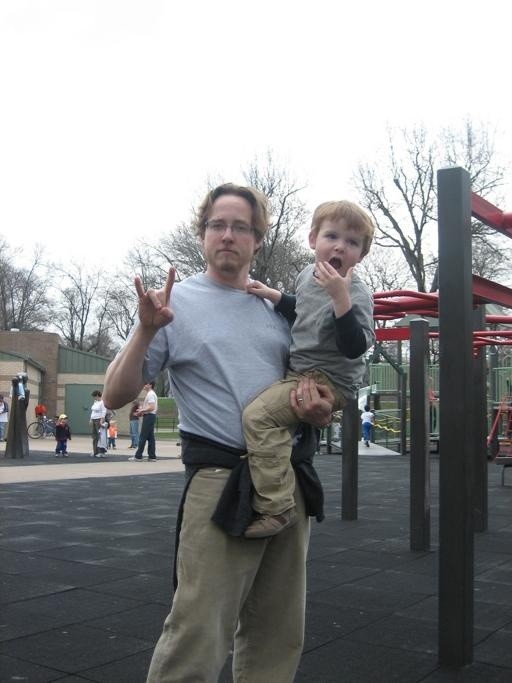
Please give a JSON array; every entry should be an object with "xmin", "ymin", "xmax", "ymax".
[
  {"xmin": 35, "ymin": 400, "xmax": 48, "ymax": 436},
  {"xmin": 128, "ymin": 399, "xmax": 142, "ymax": 449},
  {"xmin": 429, "ymin": 375, "xmax": 438, "ymax": 437},
  {"xmin": 108, "ymin": 420, "xmax": 120, "ymax": 451},
  {"xmin": 5, "ymin": 371, "xmax": 31, "ymax": 458},
  {"xmin": 9, "ymin": 376, "xmax": 26, "ymax": 402},
  {"xmin": 88, "ymin": 389, "xmax": 108, "ymax": 458},
  {"xmin": 95, "ymin": 417, "xmax": 110, "ymax": 457},
  {"xmin": 360, "ymin": 404, "xmax": 375, "ymax": 447},
  {"xmin": 242, "ymin": 199, "xmax": 378, "ymax": 540},
  {"xmin": 128, "ymin": 379, "xmax": 159, "ymax": 462},
  {"xmin": 0, "ymin": 393, "xmax": 11, "ymax": 443},
  {"xmin": 56, "ymin": 414, "xmax": 72, "ymax": 456},
  {"xmin": 101, "ymin": 181, "xmax": 326, "ymax": 682}
]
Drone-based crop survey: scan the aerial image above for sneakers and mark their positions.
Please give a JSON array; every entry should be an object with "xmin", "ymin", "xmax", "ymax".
[
  {"xmin": 128, "ymin": 457, "xmax": 157, "ymax": 462},
  {"xmin": 55, "ymin": 453, "xmax": 68, "ymax": 457},
  {"xmin": 128, "ymin": 446, "xmax": 136, "ymax": 449},
  {"xmin": 245, "ymin": 507, "xmax": 298, "ymax": 539},
  {"xmin": 96, "ymin": 453, "xmax": 106, "ymax": 458}
]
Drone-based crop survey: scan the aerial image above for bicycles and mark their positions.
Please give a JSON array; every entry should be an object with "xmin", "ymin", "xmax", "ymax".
[{"xmin": 26, "ymin": 415, "xmax": 63, "ymax": 439}]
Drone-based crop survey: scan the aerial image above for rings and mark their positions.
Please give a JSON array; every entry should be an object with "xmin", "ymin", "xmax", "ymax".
[{"xmin": 298, "ymin": 397, "xmax": 304, "ymax": 402}]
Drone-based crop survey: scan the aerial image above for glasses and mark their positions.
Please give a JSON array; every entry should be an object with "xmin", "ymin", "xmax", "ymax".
[{"xmin": 204, "ymin": 220, "xmax": 251, "ymax": 234}]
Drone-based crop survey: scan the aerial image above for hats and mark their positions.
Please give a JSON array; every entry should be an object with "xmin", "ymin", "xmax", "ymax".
[{"xmin": 59, "ymin": 414, "xmax": 68, "ymax": 419}]
